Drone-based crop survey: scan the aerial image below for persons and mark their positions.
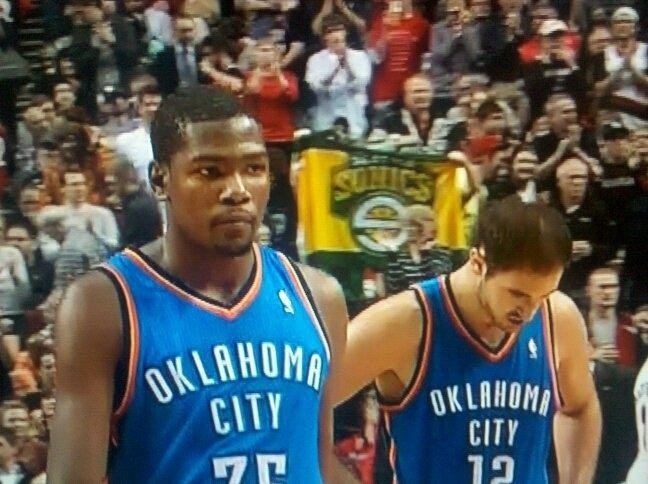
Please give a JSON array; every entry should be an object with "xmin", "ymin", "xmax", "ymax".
[{"xmin": 1, "ymin": 0, "xmax": 647, "ymax": 483}]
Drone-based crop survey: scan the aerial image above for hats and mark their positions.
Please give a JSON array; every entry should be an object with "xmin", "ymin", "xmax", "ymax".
[
  {"xmin": 537, "ymin": 17, "xmax": 570, "ymax": 35},
  {"xmin": 611, "ymin": 8, "xmax": 639, "ymax": 22}
]
